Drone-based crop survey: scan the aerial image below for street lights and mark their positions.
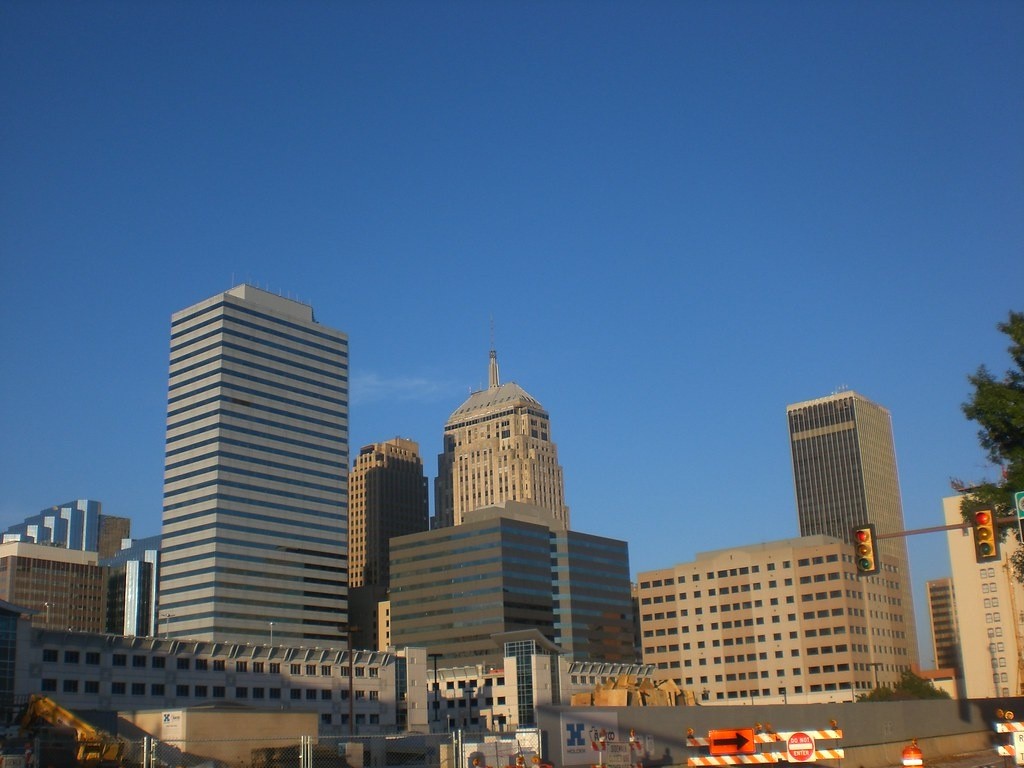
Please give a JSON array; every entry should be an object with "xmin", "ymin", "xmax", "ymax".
[
  {"xmin": 45, "ymin": 602, "xmax": 57, "ymax": 629},
  {"xmin": 270, "ymin": 622, "xmax": 275, "ymax": 646},
  {"xmin": 166, "ymin": 614, "xmax": 175, "ymax": 639}
]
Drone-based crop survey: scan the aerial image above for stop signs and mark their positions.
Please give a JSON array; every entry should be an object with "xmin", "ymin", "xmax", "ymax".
[{"xmin": 784, "ymin": 730, "xmax": 817, "ymax": 764}]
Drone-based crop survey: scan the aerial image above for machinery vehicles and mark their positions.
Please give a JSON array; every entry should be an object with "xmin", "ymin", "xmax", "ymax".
[{"xmin": 0, "ymin": 693, "xmax": 142, "ymax": 768}]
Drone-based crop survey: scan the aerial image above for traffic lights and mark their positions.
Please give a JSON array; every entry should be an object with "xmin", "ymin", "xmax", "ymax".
[
  {"xmin": 850, "ymin": 523, "xmax": 880, "ymax": 576},
  {"xmin": 971, "ymin": 507, "xmax": 1002, "ymax": 564}
]
